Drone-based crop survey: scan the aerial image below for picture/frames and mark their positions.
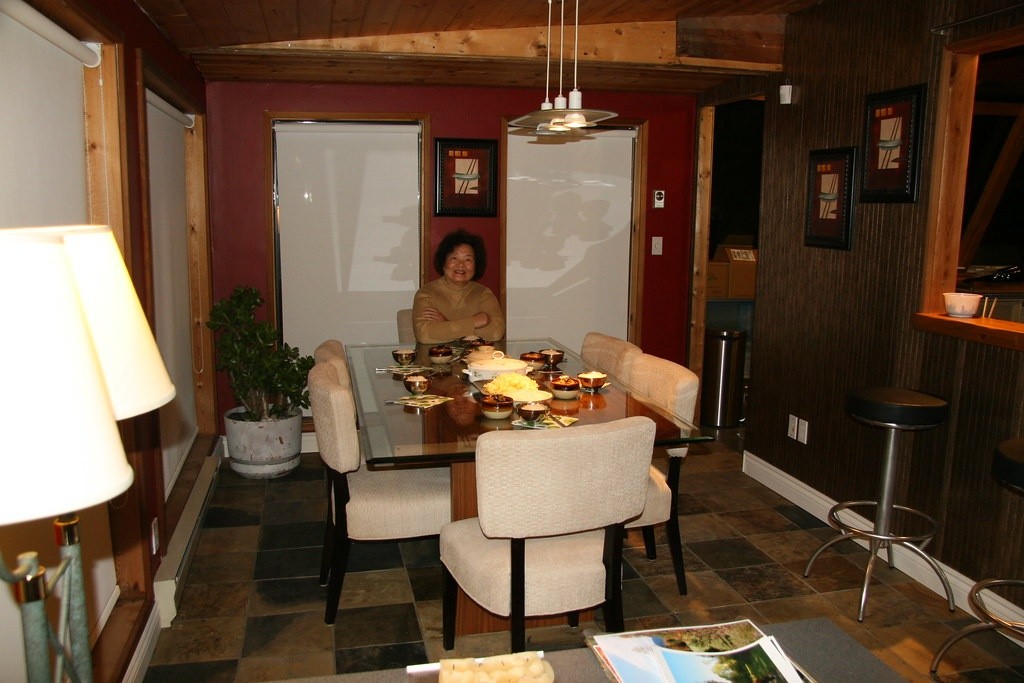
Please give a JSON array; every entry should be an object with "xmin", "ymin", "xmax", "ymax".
[
  {"xmin": 860, "ymin": 86, "xmax": 926, "ymax": 206},
  {"xmin": 803, "ymin": 146, "xmax": 855, "ymax": 252},
  {"xmin": 434, "ymin": 137, "xmax": 499, "ymax": 217}
]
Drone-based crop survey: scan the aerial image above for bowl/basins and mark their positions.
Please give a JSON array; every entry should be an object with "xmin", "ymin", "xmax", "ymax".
[
  {"xmin": 402, "ymin": 405, "xmax": 431, "ymax": 415},
  {"xmin": 550, "ymin": 378, "xmax": 580, "ymax": 400},
  {"xmin": 428, "ymin": 346, "xmax": 453, "ymax": 364},
  {"xmin": 538, "ymin": 349, "xmax": 564, "ymax": 366},
  {"xmin": 577, "ymin": 371, "xmax": 608, "ymax": 393},
  {"xmin": 480, "ymin": 396, "xmax": 514, "ymax": 420},
  {"xmin": 515, "ymin": 403, "xmax": 550, "ymax": 421},
  {"xmin": 403, "ymin": 376, "xmax": 432, "ymax": 394},
  {"xmin": 392, "ymin": 349, "xmax": 418, "ymax": 366},
  {"xmin": 520, "ymin": 352, "xmax": 545, "ymax": 371},
  {"xmin": 460, "ymin": 336, "xmax": 484, "ymax": 348},
  {"xmin": 943, "ymin": 292, "xmax": 984, "ymax": 318}
]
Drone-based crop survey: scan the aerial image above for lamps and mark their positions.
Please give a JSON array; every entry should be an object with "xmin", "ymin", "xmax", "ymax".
[
  {"xmin": 507, "ymin": 1, "xmax": 618, "ymax": 136},
  {"xmin": 0, "ymin": 225, "xmax": 176, "ymax": 683}
]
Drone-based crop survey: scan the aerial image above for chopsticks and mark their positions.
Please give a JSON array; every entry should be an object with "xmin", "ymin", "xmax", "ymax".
[{"xmin": 981, "ymin": 297, "xmax": 998, "ymax": 318}]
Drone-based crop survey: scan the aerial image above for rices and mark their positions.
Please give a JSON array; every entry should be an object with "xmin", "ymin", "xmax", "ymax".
[
  {"xmin": 540, "ymin": 350, "xmax": 563, "ymax": 354},
  {"xmin": 394, "ymin": 349, "xmax": 416, "ymax": 352},
  {"xmin": 578, "ymin": 371, "xmax": 607, "ymax": 378},
  {"xmin": 521, "ymin": 403, "xmax": 547, "ymax": 410},
  {"xmin": 405, "ymin": 375, "xmax": 429, "ymax": 381},
  {"xmin": 507, "ymin": 388, "xmax": 551, "ymax": 401}
]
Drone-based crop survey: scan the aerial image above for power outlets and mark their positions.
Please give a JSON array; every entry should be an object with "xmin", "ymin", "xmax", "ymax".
[
  {"xmin": 788, "ymin": 414, "xmax": 797, "ymax": 442},
  {"xmin": 800, "ymin": 417, "xmax": 808, "ymax": 444}
]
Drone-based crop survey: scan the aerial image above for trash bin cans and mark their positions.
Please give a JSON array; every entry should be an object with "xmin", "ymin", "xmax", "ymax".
[{"xmin": 701, "ymin": 328, "xmax": 747, "ymax": 428}]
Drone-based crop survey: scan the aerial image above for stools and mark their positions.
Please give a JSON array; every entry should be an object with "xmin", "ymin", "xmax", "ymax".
[
  {"xmin": 929, "ymin": 439, "xmax": 1024, "ymax": 673},
  {"xmin": 804, "ymin": 385, "xmax": 956, "ymax": 622}
]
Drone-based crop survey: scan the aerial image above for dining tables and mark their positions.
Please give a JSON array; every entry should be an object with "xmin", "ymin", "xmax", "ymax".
[{"xmin": 345, "ymin": 337, "xmax": 681, "ymax": 634}]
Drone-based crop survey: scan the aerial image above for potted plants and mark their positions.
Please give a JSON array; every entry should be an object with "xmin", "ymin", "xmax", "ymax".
[{"xmin": 205, "ymin": 285, "xmax": 314, "ymax": 481}]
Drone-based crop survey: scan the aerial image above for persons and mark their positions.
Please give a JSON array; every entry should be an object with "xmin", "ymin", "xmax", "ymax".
[
  {"xmin": 412, "ymin": 230, "xmax": 505, "ymax": 344},
  {"xmin": 413, "ymin": 344, "xmax": 482, "ymax": 434}
]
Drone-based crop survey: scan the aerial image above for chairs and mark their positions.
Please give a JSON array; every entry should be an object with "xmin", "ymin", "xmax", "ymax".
[{"xmin": 308, "ymin": 308, "xmax": 698, "ymax": 654}]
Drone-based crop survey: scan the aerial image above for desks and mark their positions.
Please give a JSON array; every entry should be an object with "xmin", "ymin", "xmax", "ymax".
[{"xmin": 265, "ymin": 618, "xmax": 908, "ymax": 683}]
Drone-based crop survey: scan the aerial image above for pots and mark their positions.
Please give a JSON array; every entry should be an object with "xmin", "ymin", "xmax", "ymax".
[
  {"xmin": 462, "ymin": 345, "xmax": 507, "ymax": 365},
  {"xmin": 461, "ymin": 351, "xmax": 532, "ymax": 383}
]
